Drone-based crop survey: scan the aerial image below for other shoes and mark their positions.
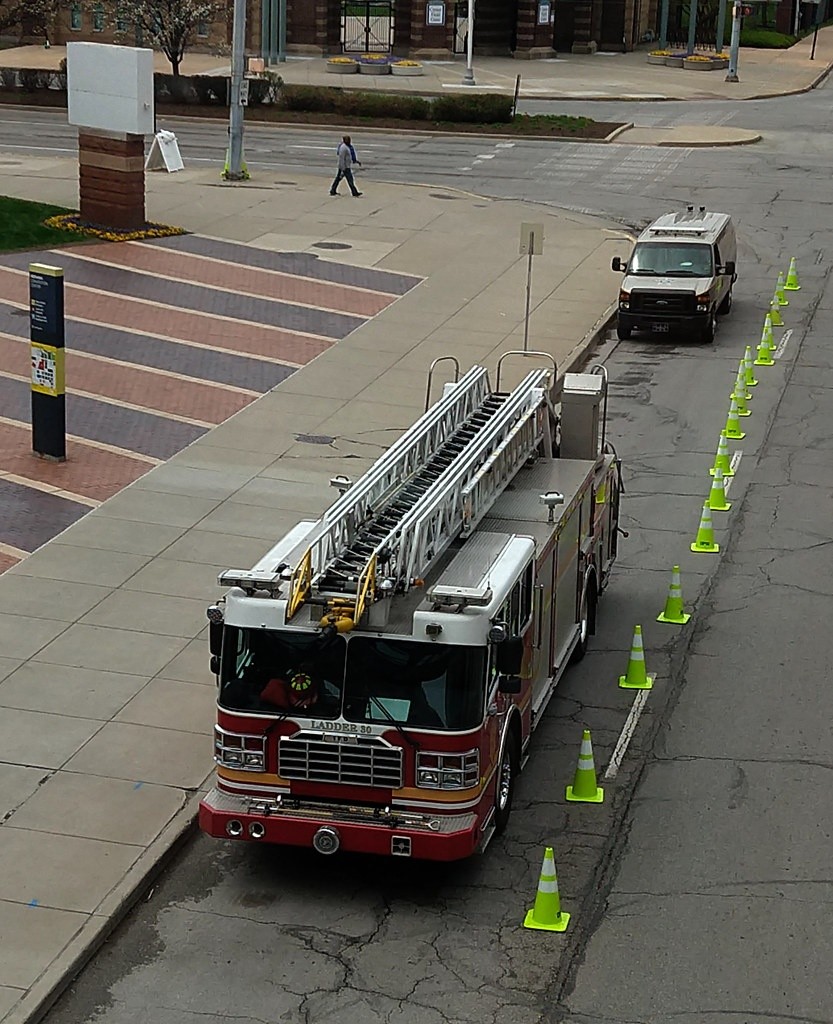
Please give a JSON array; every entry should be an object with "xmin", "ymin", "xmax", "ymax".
[
  {"xmin": 353, "ymin": 192, "xmax": 362, "ymax": 196},
  {"xmin": 330, "ymin": 189, "xmax": 337, "ymax": 195}
]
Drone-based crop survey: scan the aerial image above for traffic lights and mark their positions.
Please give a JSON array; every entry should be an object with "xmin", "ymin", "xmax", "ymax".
[{"xmin": 739, "ymin": 5, "xmax": 752, "ymax": 18}]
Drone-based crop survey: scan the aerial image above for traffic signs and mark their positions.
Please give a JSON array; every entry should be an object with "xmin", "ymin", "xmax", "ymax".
[{"xmin": 240, "ymin": 81, "xmax": 248, "ymax": 107}]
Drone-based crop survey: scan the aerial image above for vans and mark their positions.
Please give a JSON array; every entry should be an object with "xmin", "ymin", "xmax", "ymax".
[{"xmin": 611, "ymin": 205, "xmax": 740, "ymax": 341}]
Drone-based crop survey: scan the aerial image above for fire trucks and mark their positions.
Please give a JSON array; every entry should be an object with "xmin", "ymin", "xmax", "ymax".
[{"xmin": 200, "ymin": 348, "xmax": 627, "ymax": 876}]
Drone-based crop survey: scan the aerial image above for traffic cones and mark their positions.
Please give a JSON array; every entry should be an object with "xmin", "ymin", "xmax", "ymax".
[
  {"xmin": 731, "ymin": 359, "xmax": 752, "ymax": 399},
  {"xmin": 721, "ymin": 396, "xmax": 746, "ymax": 439},
  {"xmin": 690, "ymin": 499, "xmax": 719, "ymax": 553},
  {"xmin": 521, "ymin": 848, "xmax": 574, "ymax": 930},
  {"xmin": 728, "ymin": 373, "xmax": 751, "ymax": 417},
  {"xmin": 741, "ymin": 345, "xmax": 759, "ymax": 385},
  {"xmin": 706, "ymin": 462, "xmax": 732, "ymax": 512},
  {"xmin": 754, "ymin": 328, "xmax": 774, "ymax": 366},
  {"xmin": 656, "ymin": 565, "xmax": 692, "ymax": 625},
  {"xmin": 618, "ymin": 625, "xmax": 653, "ymax": 691},
  {"xmin": 567, "ymin": 730, "xmax": 604, "ymax": 804},
  {"xmin": 758, "ymin": 313, "xmax": 777, "ymax": 350},
  {"xmin": 709, "ymin": 429, "xmax": 735, "ymax": 476},
  {"xmin": 770, "ymin": 292, "xmax": 786, "ymax": 325},
  {"xmin": 769, "ymin": 273, "xmax": 789, "ymax": 304},
  {"xmin": 785, "ymin": 256, "xmax": 801, "ymax": 290}
]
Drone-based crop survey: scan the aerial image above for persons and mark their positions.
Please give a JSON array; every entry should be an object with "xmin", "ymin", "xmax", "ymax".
[{"xmin": 330, "ymin": 135, "xmax": 363, "ymax": 197}]
